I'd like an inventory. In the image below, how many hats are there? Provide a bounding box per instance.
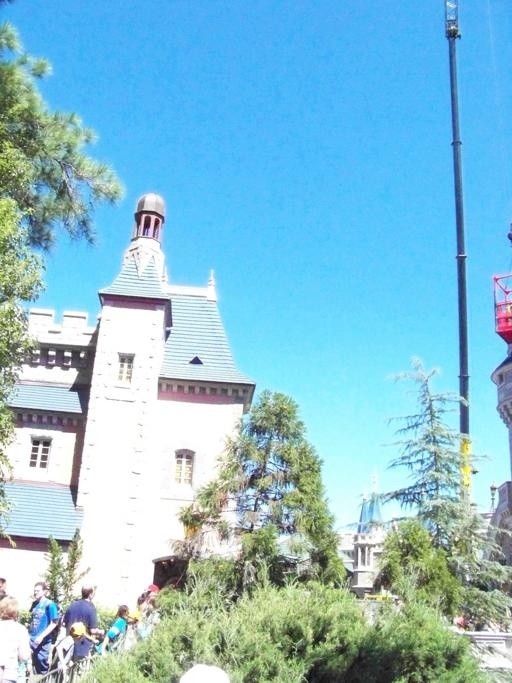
[69,623,87,637]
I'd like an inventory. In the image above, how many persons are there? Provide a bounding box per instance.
[0,574,167,683]
